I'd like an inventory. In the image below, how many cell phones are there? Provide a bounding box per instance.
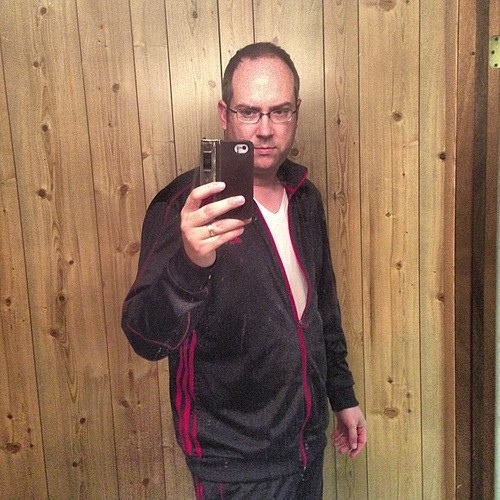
[200,137,254,221]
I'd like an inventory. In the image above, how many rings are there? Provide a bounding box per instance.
[206,222,217,238]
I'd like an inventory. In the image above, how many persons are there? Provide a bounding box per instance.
[119,42,367,499]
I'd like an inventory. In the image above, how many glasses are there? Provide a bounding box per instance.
[225,107,299,123]
